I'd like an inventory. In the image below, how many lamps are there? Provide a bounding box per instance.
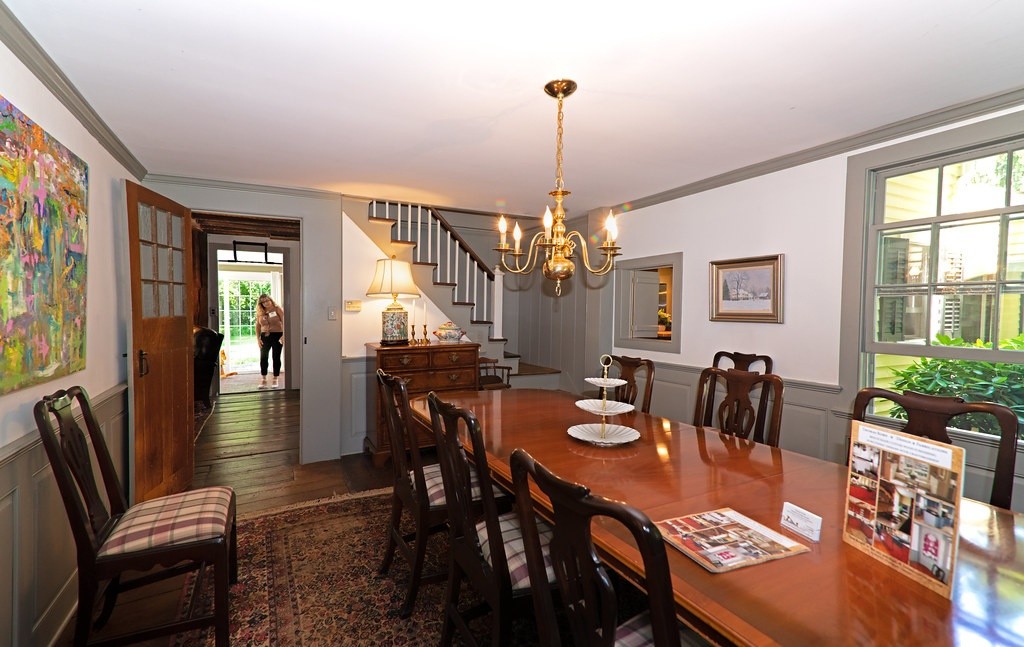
[490,79,623,299]
[366,256,421,346]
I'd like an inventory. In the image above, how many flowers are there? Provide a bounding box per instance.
[658,309,672,322]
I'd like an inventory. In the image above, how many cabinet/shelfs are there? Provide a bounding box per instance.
[365,340,479,468]
[568,356,641,447]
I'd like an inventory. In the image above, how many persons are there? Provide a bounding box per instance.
[256,294,284,389]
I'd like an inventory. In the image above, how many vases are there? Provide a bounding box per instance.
[658,324,666,332]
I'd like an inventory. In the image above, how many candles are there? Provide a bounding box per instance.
[411,299,415,325]
[423,300,427,325]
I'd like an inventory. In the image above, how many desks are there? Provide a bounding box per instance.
[402,390,1024,647]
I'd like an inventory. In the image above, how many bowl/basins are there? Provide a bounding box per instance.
[433,322,466,342]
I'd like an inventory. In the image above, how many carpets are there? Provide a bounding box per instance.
[192,400,215,443]
[173,486,492,646]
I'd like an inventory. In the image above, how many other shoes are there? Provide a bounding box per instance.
[271,379,278,388]
[258,379,268,388]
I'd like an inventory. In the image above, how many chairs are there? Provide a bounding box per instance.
[600,354,655,413]
[34,385,236,647]
[376,367,505,619]
[479,356,514,390]
[708,351,772,442]
[427,390,621,647]
[511,449,682,647]
[847,387,1020,511]
[693,368,784,449]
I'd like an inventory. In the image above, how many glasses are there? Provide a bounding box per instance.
[260,299,271,304]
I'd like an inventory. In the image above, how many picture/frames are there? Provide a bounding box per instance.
[709,253,786,324]
